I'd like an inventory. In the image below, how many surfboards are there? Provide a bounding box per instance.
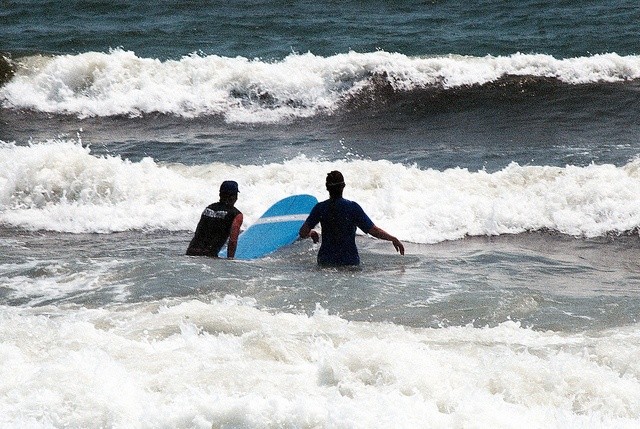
[216,194,318,260]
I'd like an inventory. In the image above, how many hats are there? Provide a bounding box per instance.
[220,181,240,197]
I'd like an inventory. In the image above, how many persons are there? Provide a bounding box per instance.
[185,181,244,259]
[299,170,405,268]
